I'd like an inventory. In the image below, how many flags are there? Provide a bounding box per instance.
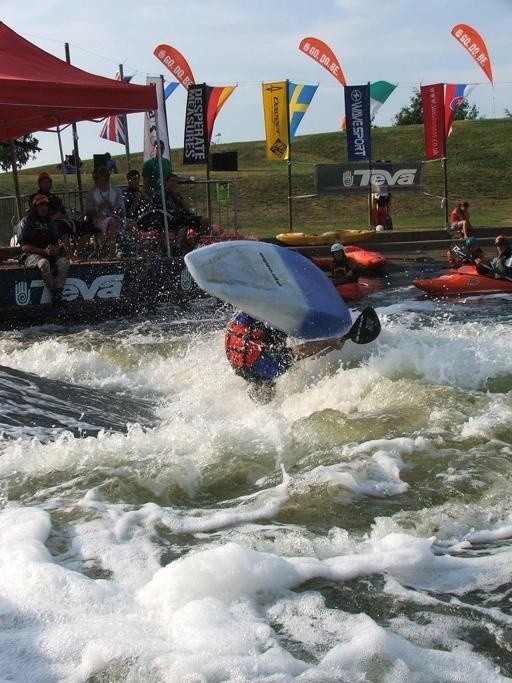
[443,83,477,140]
[99,73,132,145]
[288,80,320,144]
[342,80,398,131]
[205,85,238,145]
[164,79,180,100]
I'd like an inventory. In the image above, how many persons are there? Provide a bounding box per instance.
[224,312,344,384]
[17,193,70,309]
[371,193,392,230]
[475,234,512,282]
[67,150,83,169]
[85,139,210,260]
[326,243,358,285]
[29,172,81,264]
[447,235,485,266]
[450,201,470,239]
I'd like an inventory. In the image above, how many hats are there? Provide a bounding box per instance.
[466,236,477,247]
[330,243,345,253]
[495,234,510,247]
[32,193,49,205]
[246,380,276,406]
[38,172,52,183]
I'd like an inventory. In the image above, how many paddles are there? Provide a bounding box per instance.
[448,240,512,281]
[312,307,381,361]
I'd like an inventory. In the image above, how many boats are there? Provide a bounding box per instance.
[181,238,352,341]
[277,228,378,245]
[308,243,512,302]
[142,222,224,242]
